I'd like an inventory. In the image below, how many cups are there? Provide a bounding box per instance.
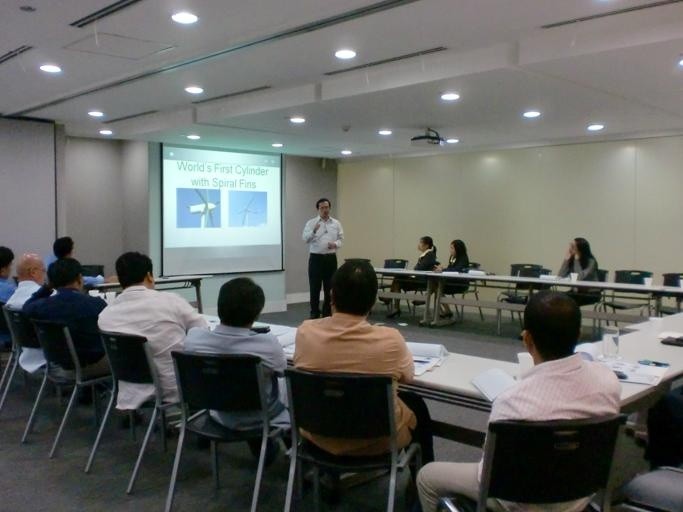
[643,277,652,286]
[680,280,683,289]
[570,272,579,281]
[87,289,116,305]
[601,325,620,358]
[517,352,532,373]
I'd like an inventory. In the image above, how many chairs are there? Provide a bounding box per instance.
[374,259,681,338]
[281,368,421,511]
[442,412,627,510]
[0,302,68,411]
[85,329,220,493]
[617,387,683,510]
[161,350,301,512]
[19,319,137,459]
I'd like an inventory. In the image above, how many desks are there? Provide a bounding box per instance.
[569,309,681,419]
[375,268,682,330]
[90,276,211,323]
[193,315,610,452]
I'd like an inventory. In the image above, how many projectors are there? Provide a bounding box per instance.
[411,136,445,146]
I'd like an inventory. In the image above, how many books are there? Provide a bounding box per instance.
[404,341,448,376]
[471,367,519,402]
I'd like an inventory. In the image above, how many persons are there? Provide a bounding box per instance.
[415,290,623,511]
[0,246,15,348]
[21,257,143,430]
[5,253,48,372]
[290,257,434,510]
[300,198,343,321]
[517,236,601,341]
[181,276,291,462]
[437,240,470,318]
[377,235,437,319]
[97,250,207,399]
[44,236,118,288]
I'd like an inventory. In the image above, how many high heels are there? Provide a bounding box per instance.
[378,297,389,305]
[439,313,453,319]
[412,300,425,305]
[386,309,400,318]
